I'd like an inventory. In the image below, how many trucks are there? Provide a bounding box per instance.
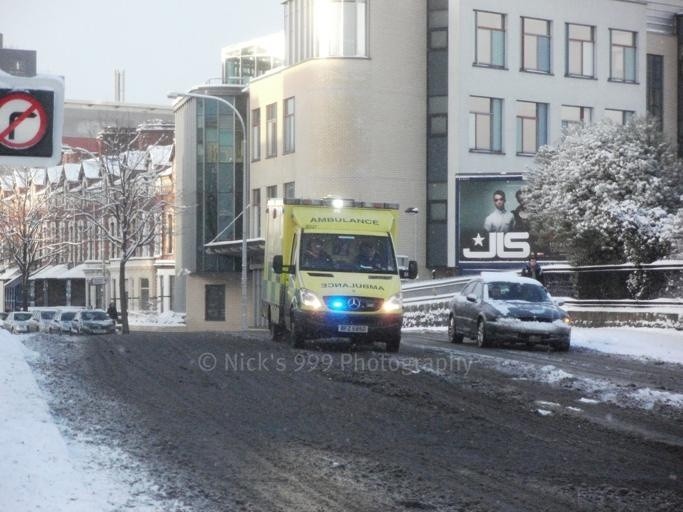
[260,197,418,353]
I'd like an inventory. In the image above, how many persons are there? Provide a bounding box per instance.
[513,187,531,232]
[522,257,548,294]
[302,239,334,271]
[353,241,385,273]
[106,301,119,334]
[484,190,516,234]
[491,286,506,299]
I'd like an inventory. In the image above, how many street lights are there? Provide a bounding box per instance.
[166,91,250,340]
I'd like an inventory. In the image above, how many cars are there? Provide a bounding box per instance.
[0,306,116,334]
[448,271,572,352]
